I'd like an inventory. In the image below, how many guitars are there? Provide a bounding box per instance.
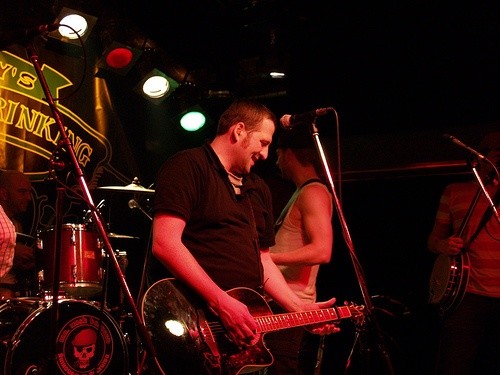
[429,173,490,311]
[142,278,364,375]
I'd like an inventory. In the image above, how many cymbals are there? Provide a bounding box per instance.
[99,181,154,192]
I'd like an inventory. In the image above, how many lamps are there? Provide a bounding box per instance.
[47,0,215,136]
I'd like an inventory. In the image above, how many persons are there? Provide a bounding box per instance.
[146,100,340,374]
[244,172,274,250]
[0,170,41,306]
[429,137,500,375]
[270,130,333,374]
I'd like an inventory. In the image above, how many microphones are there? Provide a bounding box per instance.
[15,22,59,45]
[279,107,333,131]
[128,199,152,220]
[443,133,485,160]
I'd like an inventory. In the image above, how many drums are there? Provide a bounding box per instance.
[35,223,108,295]
[1,293,132,374]
